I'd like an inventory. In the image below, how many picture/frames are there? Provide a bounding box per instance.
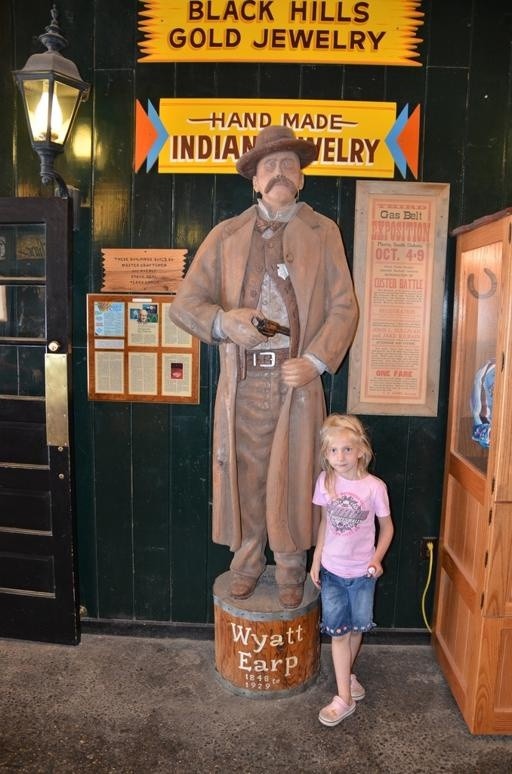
[346,179,450,417]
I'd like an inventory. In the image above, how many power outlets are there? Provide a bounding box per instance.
[420,537,437,561]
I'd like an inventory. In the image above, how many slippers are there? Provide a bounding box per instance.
[351,672,365,701]
[318,696,356,727]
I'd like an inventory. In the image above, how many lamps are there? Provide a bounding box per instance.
[11,5,92,233]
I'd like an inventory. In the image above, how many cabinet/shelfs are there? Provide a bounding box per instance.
[430,208,512,737]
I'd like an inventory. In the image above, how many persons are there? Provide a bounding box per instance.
[169,123,358,610]
[307,413,394,728]
[137,309,149,323]
